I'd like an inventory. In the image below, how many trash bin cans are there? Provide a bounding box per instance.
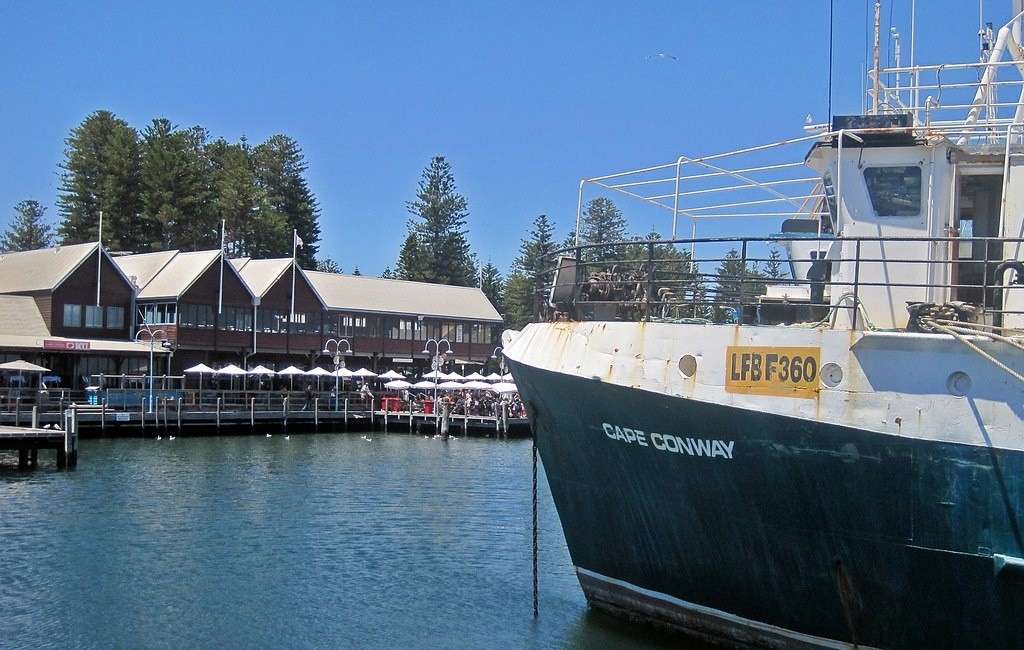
[424,400,434,414]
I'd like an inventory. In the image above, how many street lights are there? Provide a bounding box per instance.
[134,329,172,413]
[323,339,353,411]
[491,347,507,381]
[423,338,454,413]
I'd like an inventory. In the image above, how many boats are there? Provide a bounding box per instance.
[499,0,1024,650]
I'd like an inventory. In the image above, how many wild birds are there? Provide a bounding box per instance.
[645,53,679,61]
[266,434,272,437]
[485,434,489,436]
[43,424,50,430]
[361,435,372,442]
[805,114,813,125]
[157,435,161,440]
[170,435,175,440]
[424,435,429,439]
[432,434,458,440]
[284,436,290,440]
[54,424,62,431]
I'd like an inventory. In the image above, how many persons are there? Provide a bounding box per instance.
[360,382,374,411]
[399,390,525,417]
[304,385,313,411]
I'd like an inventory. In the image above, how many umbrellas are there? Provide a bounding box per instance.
[0,359,52,397]
[184,363,519,410]
